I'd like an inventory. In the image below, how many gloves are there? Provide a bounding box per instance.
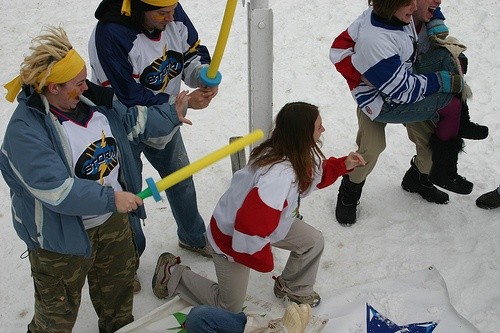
[433,71,465,96]
[427,18,449,44]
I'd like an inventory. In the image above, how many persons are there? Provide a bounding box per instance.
[87,0,218,291]
[329,0,489,227]
[476,183,500,210]
[0,23,193,332]
[152,101,366,314]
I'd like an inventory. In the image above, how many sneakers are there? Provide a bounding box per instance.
[270,276,321,309]
[129,268,141,295]
[151,252,181,300]
[176,237,213,259]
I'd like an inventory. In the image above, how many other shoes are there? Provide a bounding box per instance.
[474,185,500,211]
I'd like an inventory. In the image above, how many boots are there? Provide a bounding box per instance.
[241,302,312,333]
[400,154,449,205]
[335,174,367,228]
[429,137,474,195]
[456,92,489,140]
